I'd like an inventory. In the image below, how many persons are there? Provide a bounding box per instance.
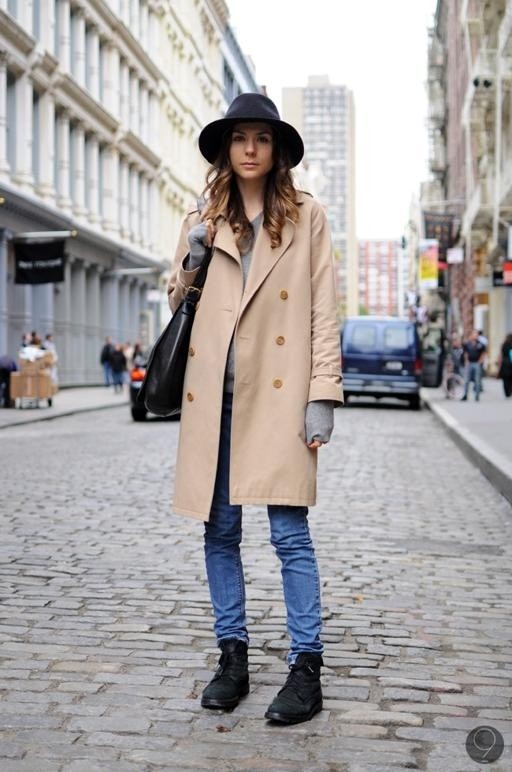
[99,335,147,395]
[164,90,347,727]
[441,328,489,402]
[0,327,59,408]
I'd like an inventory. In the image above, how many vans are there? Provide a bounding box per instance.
[335,315,447,410]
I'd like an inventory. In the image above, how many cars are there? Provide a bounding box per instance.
[130,339,182,425]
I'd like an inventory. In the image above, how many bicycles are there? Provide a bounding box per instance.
[439,336,466,401]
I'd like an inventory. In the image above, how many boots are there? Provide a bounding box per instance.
[264,651,323,723]
[200,639,249,709]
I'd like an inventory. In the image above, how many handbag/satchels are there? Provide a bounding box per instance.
[136,298,196,417]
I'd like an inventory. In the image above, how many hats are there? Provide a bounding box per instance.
[199,93,304,170]
